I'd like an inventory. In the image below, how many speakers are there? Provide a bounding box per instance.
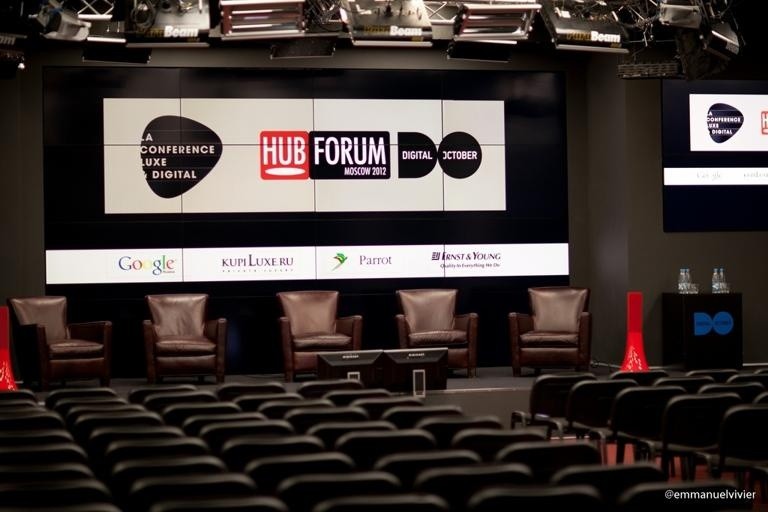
[316,349,384,388]
[384,347,450,392]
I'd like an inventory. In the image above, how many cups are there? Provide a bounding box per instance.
[678,283,729,294]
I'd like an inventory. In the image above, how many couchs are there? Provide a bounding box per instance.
[275,291,364,382]
[143,294,228,384]
[508,287,592,375]
[395,288,479,377]
[5,296,114,390]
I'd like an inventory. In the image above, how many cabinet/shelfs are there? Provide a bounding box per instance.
[661,292,743,370]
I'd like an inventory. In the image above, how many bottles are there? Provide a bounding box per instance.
[678,268,725,294]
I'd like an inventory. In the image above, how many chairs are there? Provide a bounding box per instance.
[511,369,768,501]
